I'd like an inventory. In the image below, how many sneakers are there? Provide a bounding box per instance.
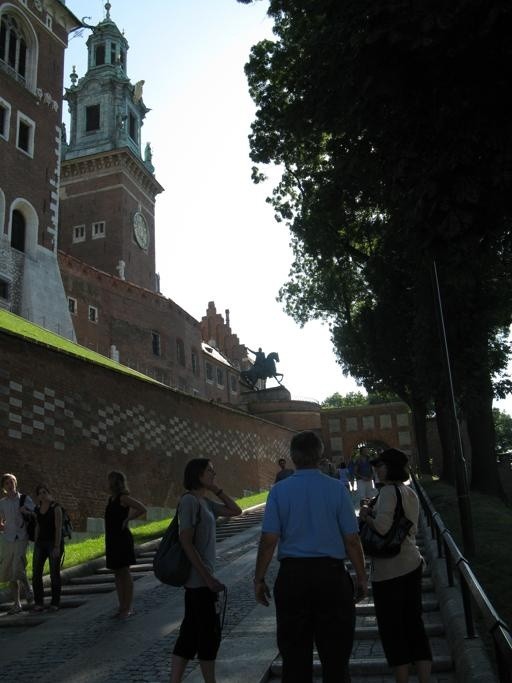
[6,584,59,615]
[110,608,137,621]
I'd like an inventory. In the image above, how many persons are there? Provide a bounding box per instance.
[253,429,367,683]
[273,456,296,485]
[31,484,64,612]
[105,469,148,619]
[320,444,385,510]
[357,447,432,683]
[1,473,35,613]
[170,458,242,683]
[246,346,266,367]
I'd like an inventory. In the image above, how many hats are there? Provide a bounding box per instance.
[370,448,408,467]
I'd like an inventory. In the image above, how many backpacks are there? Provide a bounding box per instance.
[19,493,40,542]
[51,503,73,540]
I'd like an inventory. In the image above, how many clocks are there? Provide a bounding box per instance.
[133,212,150,249]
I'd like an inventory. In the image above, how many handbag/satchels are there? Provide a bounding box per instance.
[153,514,193,587]
[357,497,414,559]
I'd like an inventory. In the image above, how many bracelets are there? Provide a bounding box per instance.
[253,577,264,584]
[30,511,33,516]
[55,542,60,547]
